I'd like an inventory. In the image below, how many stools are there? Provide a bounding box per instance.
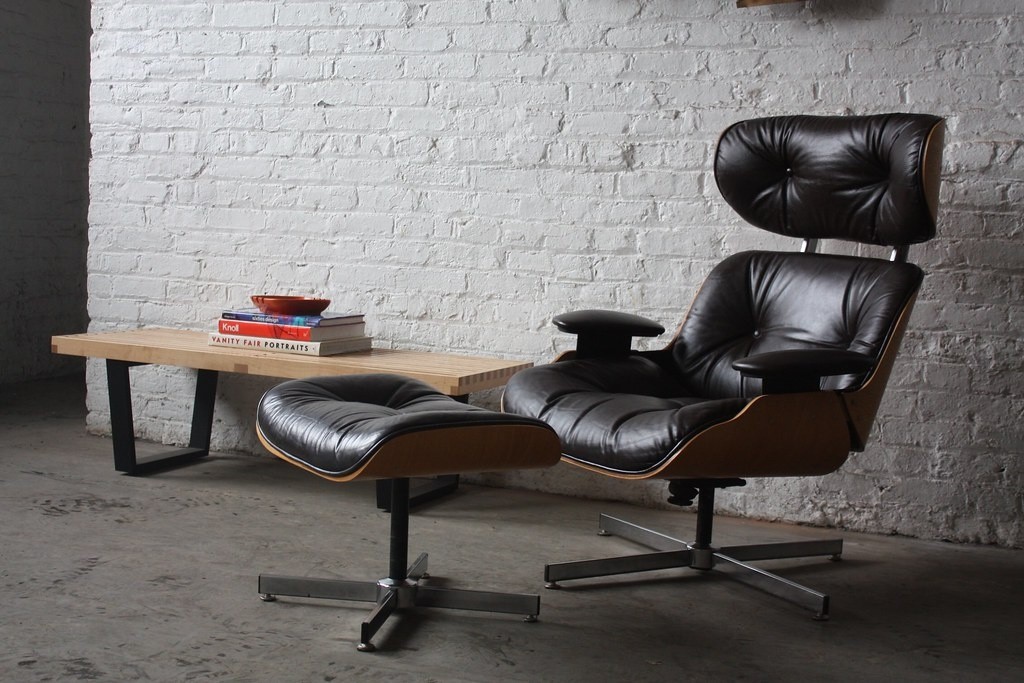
[255,372,561,650]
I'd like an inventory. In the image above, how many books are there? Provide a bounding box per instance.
[209,310,373,356]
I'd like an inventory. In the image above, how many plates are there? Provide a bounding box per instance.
[252,295,331,315]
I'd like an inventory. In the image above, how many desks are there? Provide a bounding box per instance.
[52,328,534,510]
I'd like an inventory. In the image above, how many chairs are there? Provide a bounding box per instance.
[501,112,945,620]
